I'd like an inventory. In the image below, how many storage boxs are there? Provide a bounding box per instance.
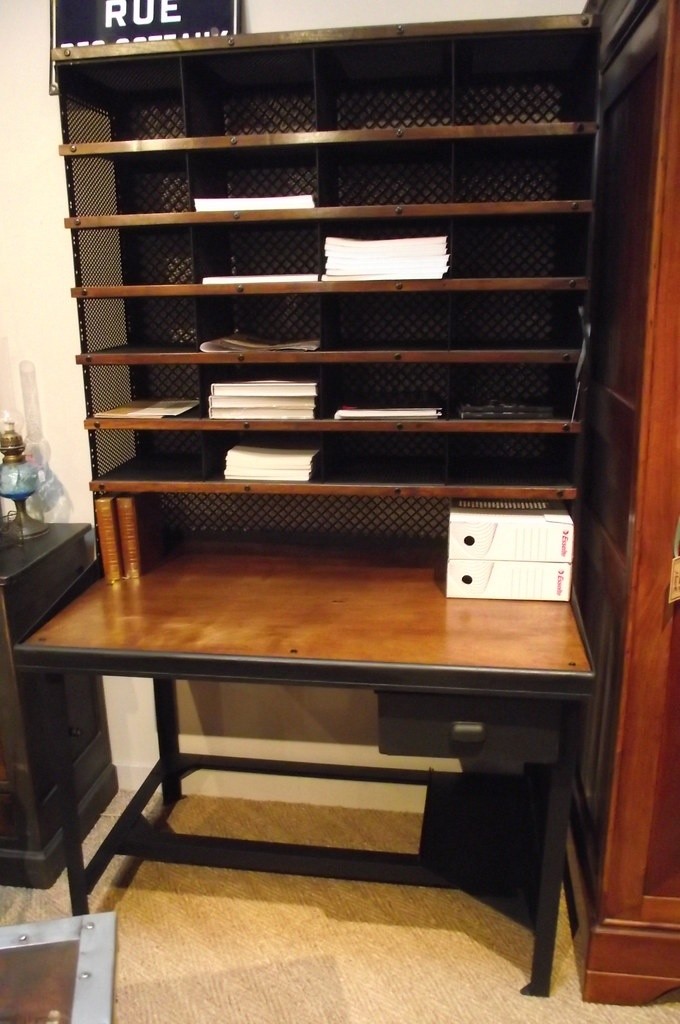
[447,501,574,601]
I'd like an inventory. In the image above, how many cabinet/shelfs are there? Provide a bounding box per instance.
[0,517,119,891]
[561,1,680,1008]
[50,12,602,546]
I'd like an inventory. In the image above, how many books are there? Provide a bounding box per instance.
[223,443,322,482]
[194,195,314,212]
[334,406,443,420]
[93,398,201,419]
[207,378,318,419]
[321,236,451,281]
[202,274,319,284]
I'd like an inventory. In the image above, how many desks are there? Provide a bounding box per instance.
[13,532,598,998]
